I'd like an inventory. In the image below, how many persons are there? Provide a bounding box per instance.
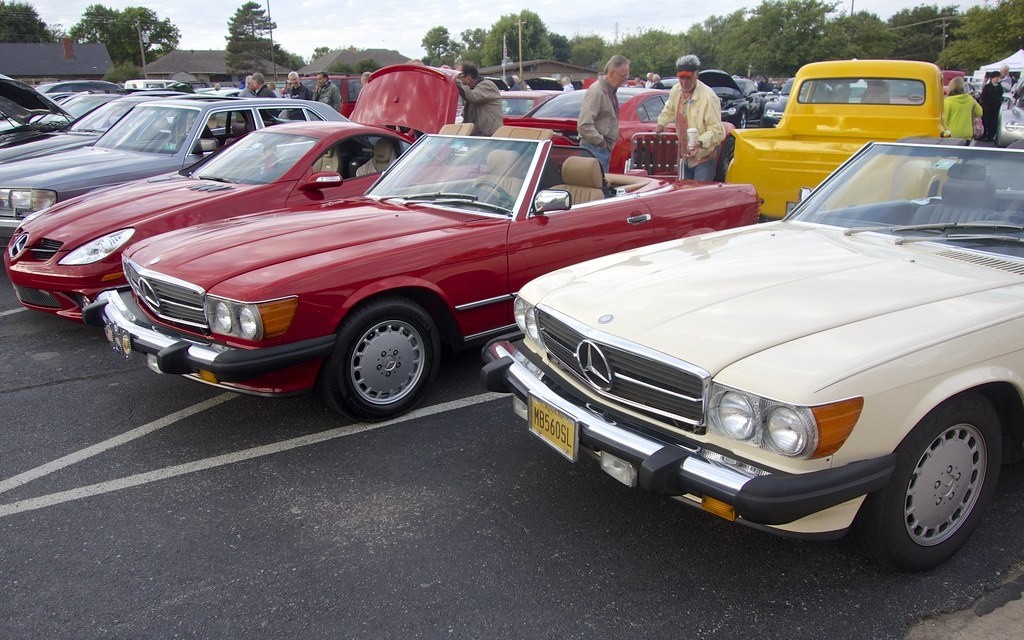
[980,66,1024,141]
[653,55,725,181]
[215,83,220,90]
[442,63,502,164]
[757,77,783,92]
[861,79,890,103]
[506,75,528,114]
[943,77,982,145]
[238,73,277,98]
[635,73,664,90]
[361,59,422,137]
[312,72,341,112]
[285,72,312,100]
[577,55,629,172]
[562,77,574,91]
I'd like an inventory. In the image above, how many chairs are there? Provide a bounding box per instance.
[356,140,396,179]
[312,139,342,177]
[226,122,245,144]
[201,124,219,149]
[473,152,522,201]
[913,174,997,234]
[546,155,606,206]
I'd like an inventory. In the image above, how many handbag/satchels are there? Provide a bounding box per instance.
[973,117,984,140]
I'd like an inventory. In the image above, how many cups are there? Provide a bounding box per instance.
[687,127,698,150]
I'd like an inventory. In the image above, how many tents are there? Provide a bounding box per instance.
[980,49,1024,79]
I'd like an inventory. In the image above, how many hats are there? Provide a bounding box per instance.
[675,65,699,77]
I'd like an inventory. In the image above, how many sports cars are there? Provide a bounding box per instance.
[81,134,764,425]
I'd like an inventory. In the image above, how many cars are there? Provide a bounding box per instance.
[478,136,1024,572]
[454,60,1024,220]
[0,64,477,326]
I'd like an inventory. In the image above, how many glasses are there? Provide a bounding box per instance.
[614,71,630,80]
